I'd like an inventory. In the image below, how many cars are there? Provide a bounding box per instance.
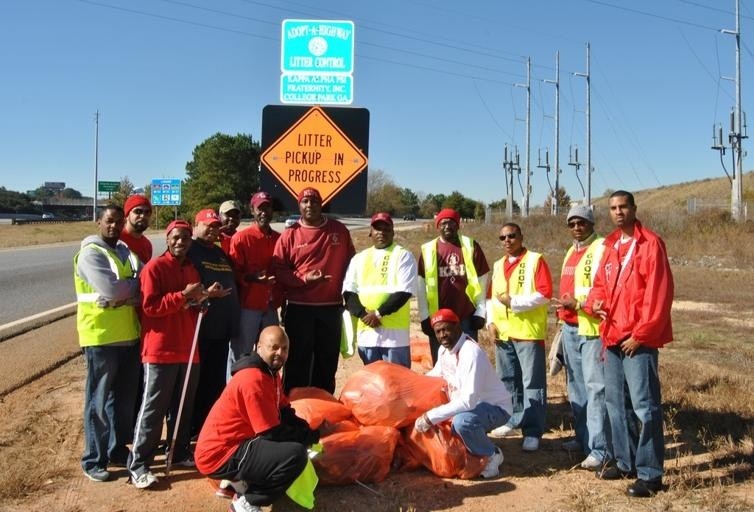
[42,212,53,219]
[285,215,300,227]
[404,213,416,221]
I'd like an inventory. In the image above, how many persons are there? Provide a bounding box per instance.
[413,306,515,480]
[413,209,492,379]
[274,184,356,397]
[580,190,675,497]
[63,190,284,496]
[486,225,553,453]
[549,205,615,470]
[340,210,420,372]
[192,322,310,512]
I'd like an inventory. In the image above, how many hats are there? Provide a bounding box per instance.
[435,207,460,229]
[166,220,193,236]
[123,194,152,217]
[298,187,322,208]
[251,192,272,207]
[219,200,241,214]
[431,308,459,328]
[566,205,595,225]
[371,212,393,225]
[195,208,222,227]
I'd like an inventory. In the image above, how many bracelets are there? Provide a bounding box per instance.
[375,308,383,321]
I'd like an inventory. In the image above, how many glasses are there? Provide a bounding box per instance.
[568,220,585,228]
[441,220,457,226]
[133,208,151,214]
[499,233,516,241]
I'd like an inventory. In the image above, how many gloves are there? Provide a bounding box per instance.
[415,415,431,433]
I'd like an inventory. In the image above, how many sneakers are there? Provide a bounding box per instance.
[167,450,195,467]
[491,425,515,436]
[216,479,249,498]
[226,493,263,512]
[628,477,663,497]
[581,455,602,470]
[522,436,539,451]
[562,440,580,451]
[108,458,127,467]
[83,465,110,482]
[602,466,632,480]
[131,470,159,489]
[480,446,504,479]
